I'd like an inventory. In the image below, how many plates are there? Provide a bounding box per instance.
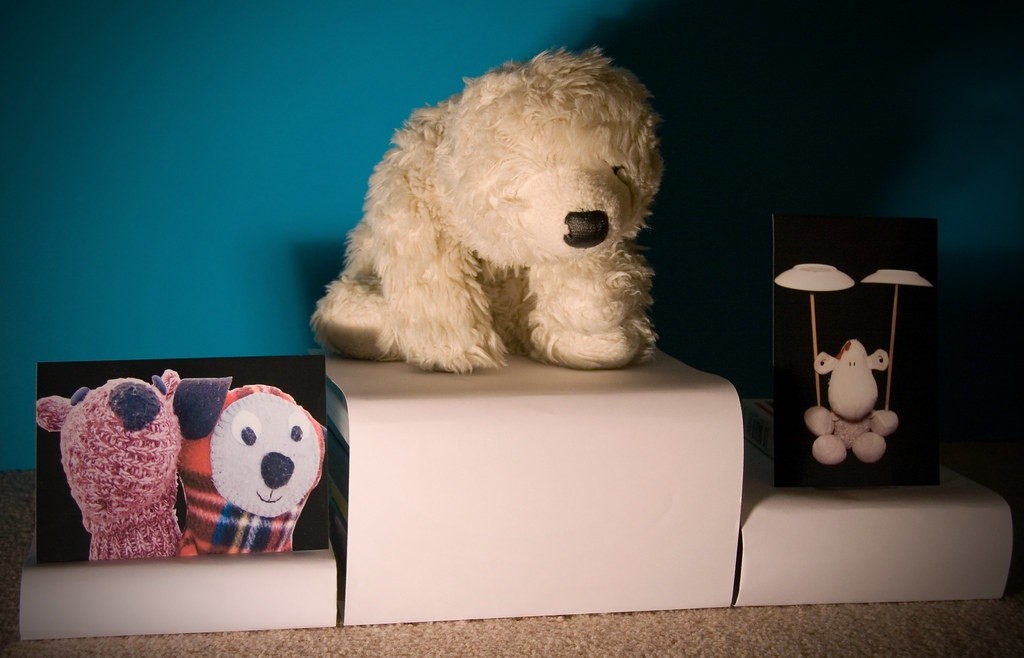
[774,263,855,292]
[860,269,933,287]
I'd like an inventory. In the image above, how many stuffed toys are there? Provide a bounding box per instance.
[310,44,666,374]
